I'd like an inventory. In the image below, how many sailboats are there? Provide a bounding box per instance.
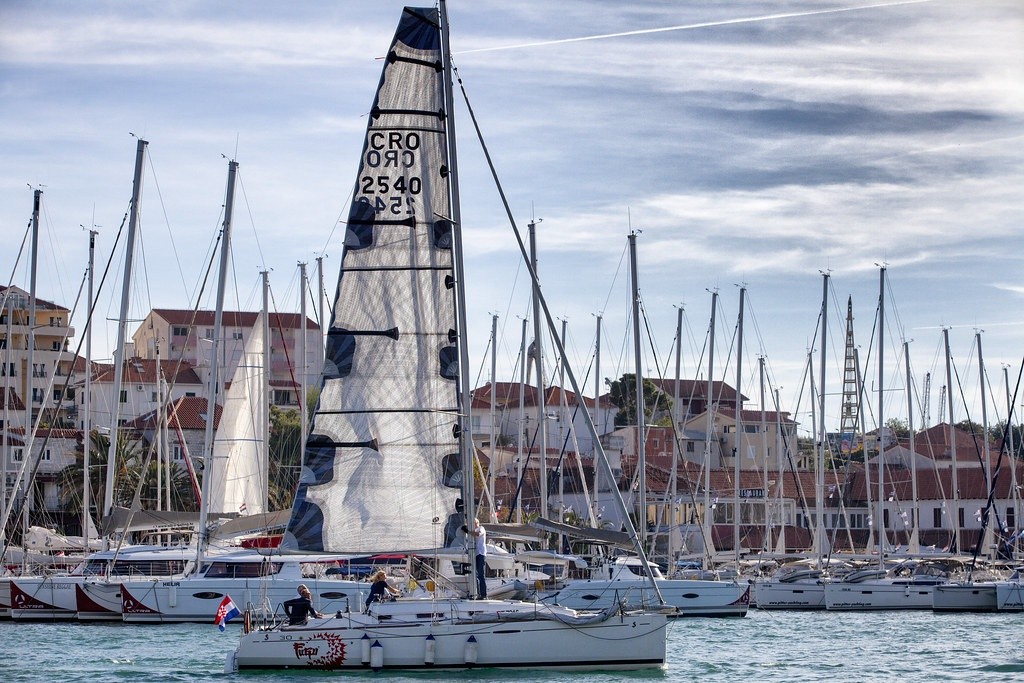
[0,0,1023,669]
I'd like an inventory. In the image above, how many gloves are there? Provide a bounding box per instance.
[462,526,468,533]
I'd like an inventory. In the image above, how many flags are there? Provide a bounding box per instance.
[214,595,239,633]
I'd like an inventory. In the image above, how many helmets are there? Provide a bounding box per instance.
[300,589,310,595]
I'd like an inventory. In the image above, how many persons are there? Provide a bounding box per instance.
[468,517,488,600]
[365,571,401,610]
[283,585,324,627]
